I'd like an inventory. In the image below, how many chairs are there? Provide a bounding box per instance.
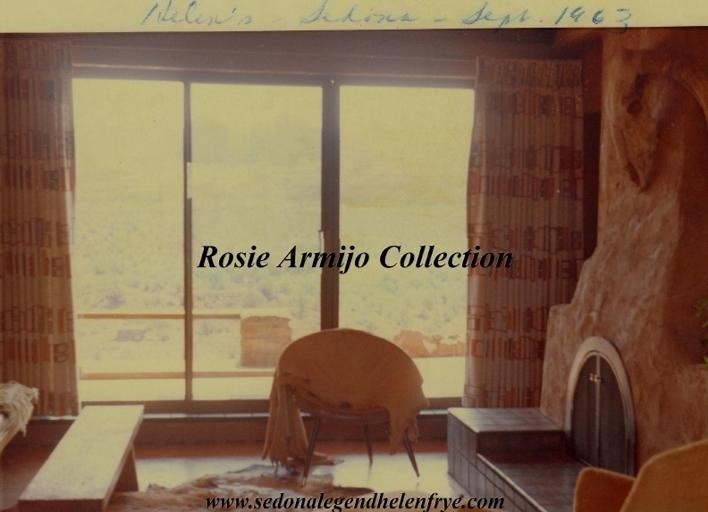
[262,327,427,477]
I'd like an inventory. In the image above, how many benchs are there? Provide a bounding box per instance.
[447,407,578,512]
[16,404,146,512]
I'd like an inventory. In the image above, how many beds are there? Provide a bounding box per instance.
[1,380,41,453]
